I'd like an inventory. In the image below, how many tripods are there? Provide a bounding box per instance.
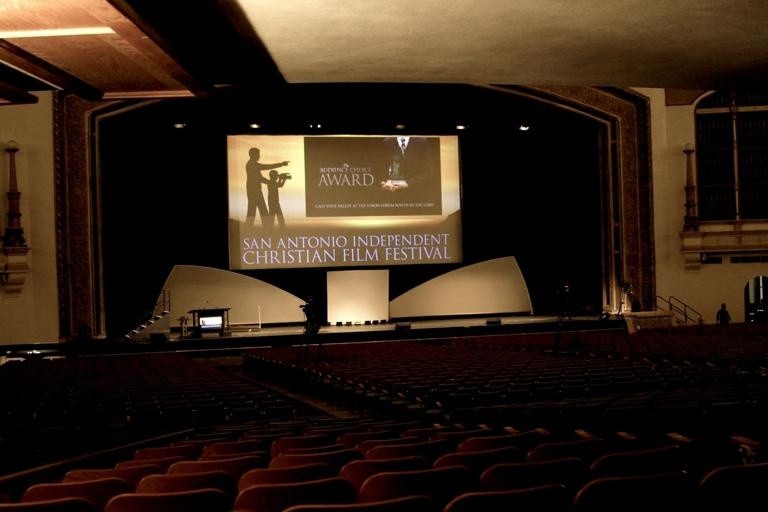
[295,324,330,368]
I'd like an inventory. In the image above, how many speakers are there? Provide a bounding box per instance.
[487,318,501,324]
[395,322,411,329]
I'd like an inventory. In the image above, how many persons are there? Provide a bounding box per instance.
[716,303,732,324]
[299,295,321,334]
[245,149,292,231]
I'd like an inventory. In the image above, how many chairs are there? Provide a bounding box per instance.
[0,323,767,512]
